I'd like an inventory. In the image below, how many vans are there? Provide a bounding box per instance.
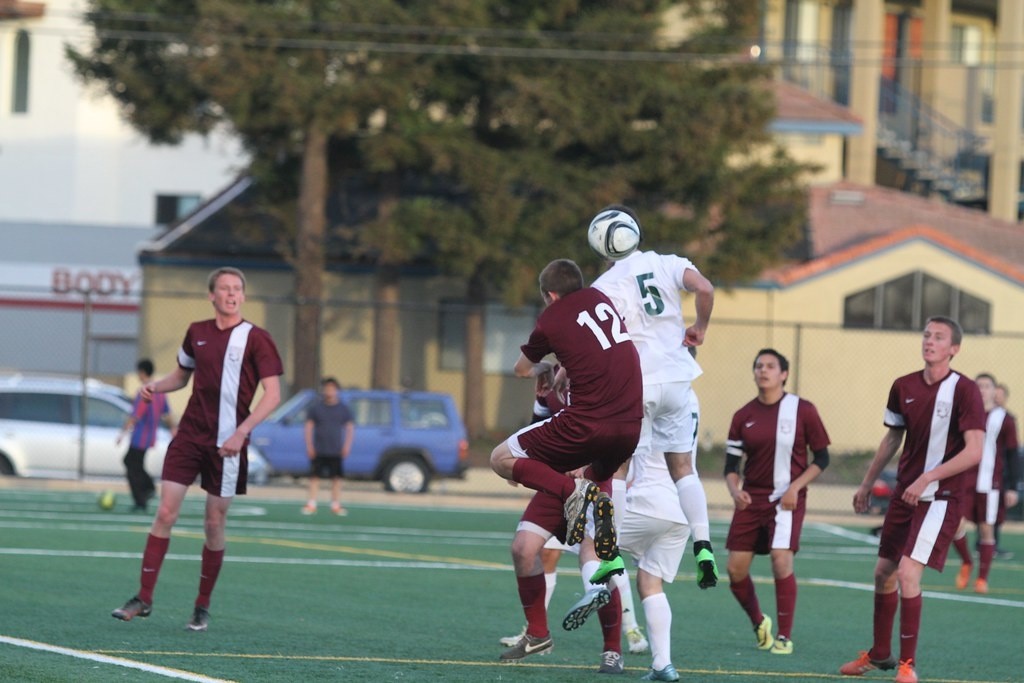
[246,385,472,496]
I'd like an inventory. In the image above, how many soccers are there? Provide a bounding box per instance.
[586,209,641,262]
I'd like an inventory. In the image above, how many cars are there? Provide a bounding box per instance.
[0,367,174,483]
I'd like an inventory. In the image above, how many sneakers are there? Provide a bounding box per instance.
[597,650,624,674]
[896,658,917,683]
[500,624,528,646]
[754,614,774,650]
[186,605,210,630]
[499,630,554,662]
[111,596,153,622]
[564,478,600,547]
[592,492,617,560]
[975,579,986,593]
[695,548,719,588]
[590,555,625,584]
[957,565,972,589]
[639,665,679,682]
[623,626,649,654]
[562,587,611,631]
[770,635,793,654]
[840,648,896,676]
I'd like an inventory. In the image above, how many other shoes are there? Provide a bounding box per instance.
[300,501,317,514]
[330,504,347,515]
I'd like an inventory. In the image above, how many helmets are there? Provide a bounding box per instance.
[587,210,640,262]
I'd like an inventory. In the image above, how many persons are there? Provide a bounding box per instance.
[500,344,700,680]
[112,266,283,628]
[590,206,717,593]
[305,379,354,517]
[725,349,831,656]
[490,259,644,561]
[953,375,1021,593]
[840,317,986,683]
[117,360,177,511]
